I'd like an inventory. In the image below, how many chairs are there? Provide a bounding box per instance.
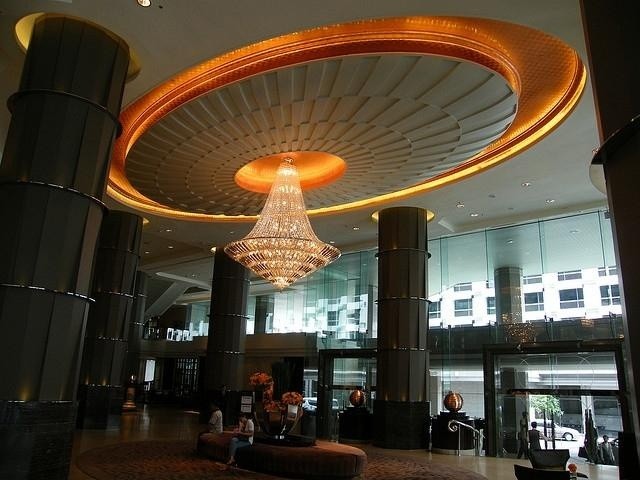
[514,448,588,480]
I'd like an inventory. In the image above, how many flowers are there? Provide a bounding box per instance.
[249,371,303,413]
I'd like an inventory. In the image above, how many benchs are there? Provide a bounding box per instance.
[198,430,367,479]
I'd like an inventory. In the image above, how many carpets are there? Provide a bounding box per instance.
[76,440,488,480]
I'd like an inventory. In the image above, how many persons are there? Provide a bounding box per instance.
[516,410,529,460]
[526,421,550,451]
[582,419,599,465]
[599,434,616,465]
[194,403,224,448]
[225,412,255,465]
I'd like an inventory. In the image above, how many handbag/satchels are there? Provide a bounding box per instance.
[237,422,251,443]
[578,446,588,459]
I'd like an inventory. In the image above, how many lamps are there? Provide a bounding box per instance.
[224,158,341,293]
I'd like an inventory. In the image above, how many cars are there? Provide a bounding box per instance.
[531,419,577,441]
[302,397,317,411]
[332,399,340,409]
